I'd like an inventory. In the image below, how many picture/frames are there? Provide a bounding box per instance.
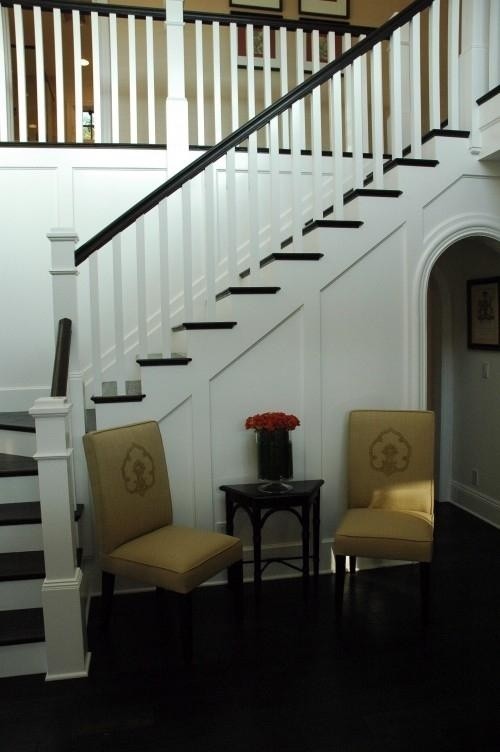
[466,276,499,352]
[228,1,352,79]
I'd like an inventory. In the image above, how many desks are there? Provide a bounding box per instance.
[216,478,326,620]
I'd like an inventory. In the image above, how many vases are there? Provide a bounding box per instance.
[257,442,296,494]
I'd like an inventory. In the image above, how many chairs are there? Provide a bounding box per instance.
[332,406,441,650]
[80,420,246,668]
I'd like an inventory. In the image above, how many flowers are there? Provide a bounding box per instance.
[244,410,302,446]
